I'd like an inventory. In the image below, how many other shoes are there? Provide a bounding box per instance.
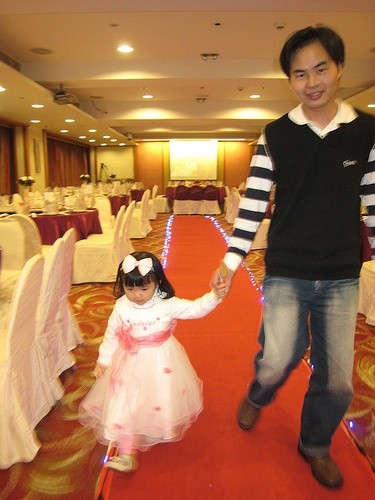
[104,453,138,474]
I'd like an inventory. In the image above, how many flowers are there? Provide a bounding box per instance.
[19,176,35,186]
[80,174,91,180]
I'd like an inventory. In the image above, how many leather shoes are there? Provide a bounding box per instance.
[238,396,260,431]
[298,440,343,488]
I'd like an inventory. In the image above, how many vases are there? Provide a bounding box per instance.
[83,179,87,187]
[22,186,28,197]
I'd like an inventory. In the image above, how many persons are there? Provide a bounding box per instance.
[211,26,375,488]
[78,250,230,472]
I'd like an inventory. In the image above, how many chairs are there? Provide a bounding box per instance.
[190,185,203,215]
[357,259,375,325]
[2,214,88,470]
[203,185,222,215]
[251,217,272,251]
[173,185,192,215]
[224,181,246,224]
[1,182,157,284]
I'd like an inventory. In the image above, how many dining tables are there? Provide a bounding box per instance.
[131,189,146,202]
[31,206,103,245]
[107,194,130,216]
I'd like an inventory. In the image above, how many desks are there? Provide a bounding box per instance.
[166,185,227,203]
